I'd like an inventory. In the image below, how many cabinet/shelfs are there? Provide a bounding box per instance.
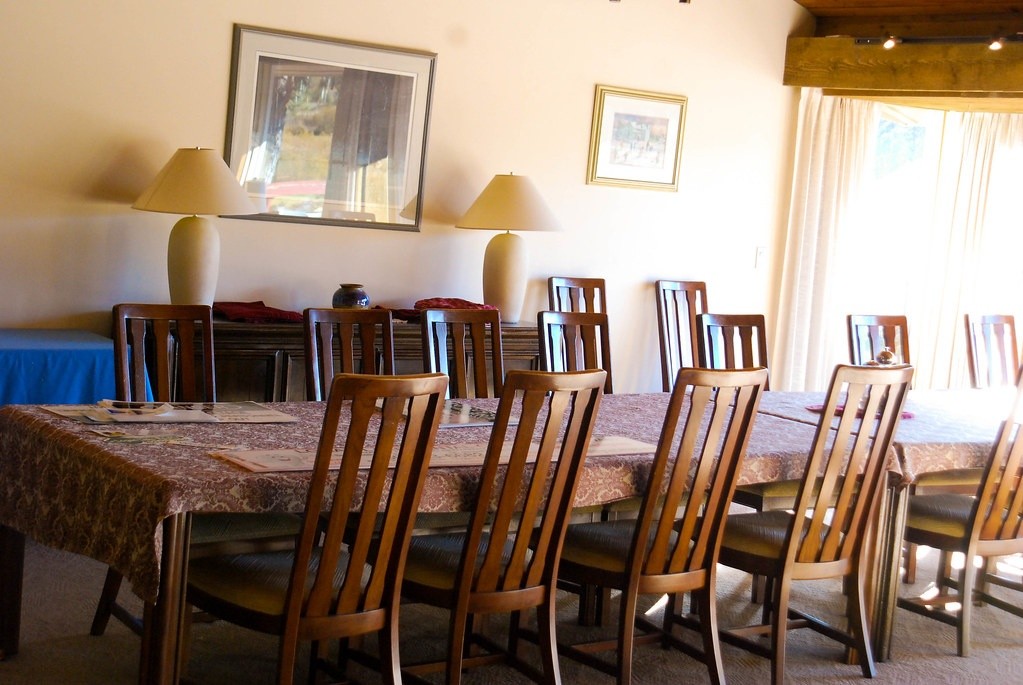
[121,322,541,402]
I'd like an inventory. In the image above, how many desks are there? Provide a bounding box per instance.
[0,401,903,685]
[0,330,154,403]
[686,391,1022,657]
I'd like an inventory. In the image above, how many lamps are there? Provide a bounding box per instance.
[133,148,261,315]
[455,174,553,325]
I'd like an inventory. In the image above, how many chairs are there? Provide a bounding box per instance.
[546,276,608,313]
[185,373,449,685]
[697,314,771,392]
[415,370,608,685]
[847,315,910,365]
[722,364,914,685]
[422,308,506,401]
[555,368,769,685]
[963,314,1021,388]
[91,304,216,641]
[900,369,1023,658]
[536,312,613,393]
[303,307,397,402]
[655,280,709,392]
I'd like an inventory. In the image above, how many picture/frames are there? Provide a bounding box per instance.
[585,84,689,192]
[222,20,439,234]
